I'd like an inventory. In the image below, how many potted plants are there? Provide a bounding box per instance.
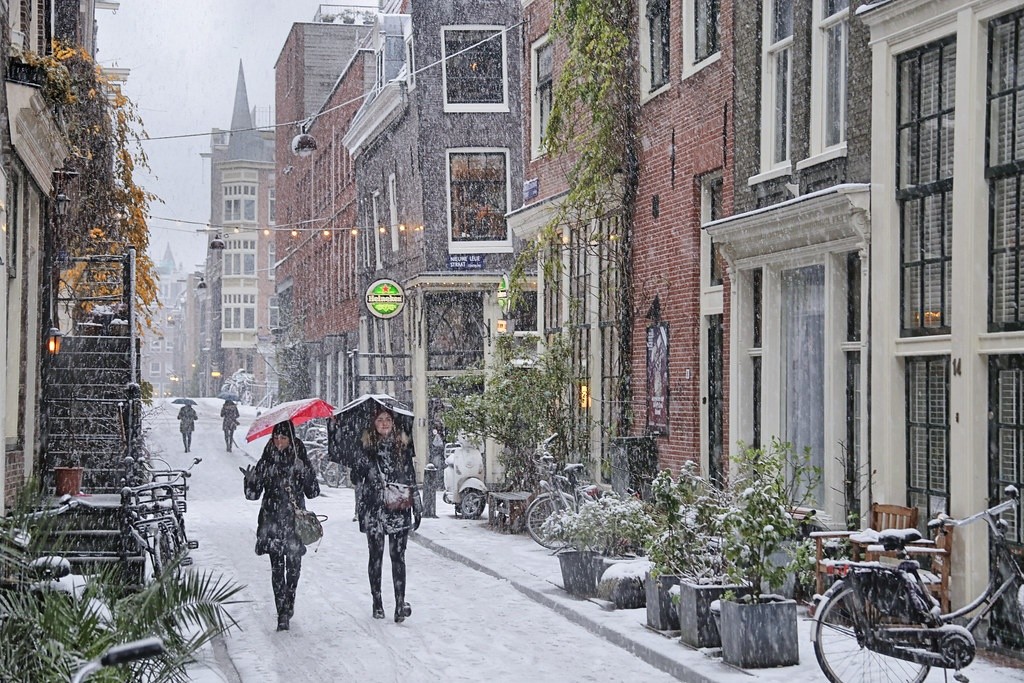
[320,9,376,24]
[538,435,820,670]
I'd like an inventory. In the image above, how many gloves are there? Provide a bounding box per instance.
[239,464,257,481]
[294,458,304,471]
[412,513,422,531]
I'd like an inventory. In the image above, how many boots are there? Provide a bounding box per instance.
[371,591,385,618]
[394,591,412,623]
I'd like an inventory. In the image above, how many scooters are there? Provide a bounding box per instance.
[441,429,488,520]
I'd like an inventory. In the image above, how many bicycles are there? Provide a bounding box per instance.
[4,494,95,615]
[106,454,203,602]
[802,486,1024,683]
[521,432,606,556]
[296,417,353,489]
[240,384,257,406]
[69,637,164,683]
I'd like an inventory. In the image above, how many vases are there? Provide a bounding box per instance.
[54,465,84,495]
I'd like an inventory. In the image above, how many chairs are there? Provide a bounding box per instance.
[859,513,953,626]
[807,501,919,626]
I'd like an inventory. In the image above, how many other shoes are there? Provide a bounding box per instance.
[278,611,294,631]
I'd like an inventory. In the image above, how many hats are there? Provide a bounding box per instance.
[273,420,295,438]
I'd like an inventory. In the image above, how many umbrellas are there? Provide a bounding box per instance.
[218,392,241,402]
[172,398,198,406]
[245,396,336,460]
[326,392,417,470]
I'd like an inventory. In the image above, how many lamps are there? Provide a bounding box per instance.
[54,182,71,221]
[196,277,207,289]
[292,123,317,157]
[42,318,64,363]
[208,235,225,249]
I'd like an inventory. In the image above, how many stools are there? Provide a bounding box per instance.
[488,491,534,534]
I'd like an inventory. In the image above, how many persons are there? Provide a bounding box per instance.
[220,399,239,453]
[239,420,321,633]
[350,408,423,623]
[177,405,198,453]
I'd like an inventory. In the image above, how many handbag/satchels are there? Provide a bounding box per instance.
[296,512,323,545]
[384,483,411,510]
